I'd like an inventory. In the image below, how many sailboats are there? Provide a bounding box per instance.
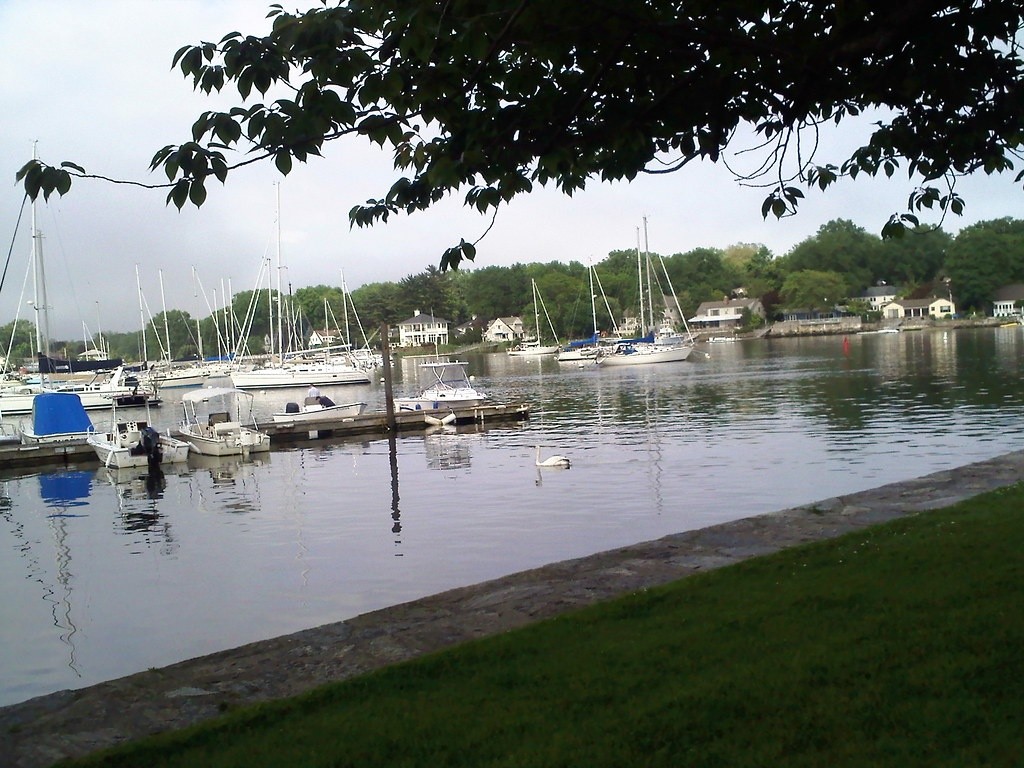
[505,278,561,356]
[0,138,394,447]
[558,216,694,365]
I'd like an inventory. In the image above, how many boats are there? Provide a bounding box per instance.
[705,335,737,343]
[271,396,369,422]
[177,385,272,457]
[86,394,189,469]
[394,305,486,413]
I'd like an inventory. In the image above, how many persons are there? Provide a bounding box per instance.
[315,396,336,409]
[308,384,320,397]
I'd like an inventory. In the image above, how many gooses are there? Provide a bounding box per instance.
[535,465,571,484]
[534,445,571,465]
